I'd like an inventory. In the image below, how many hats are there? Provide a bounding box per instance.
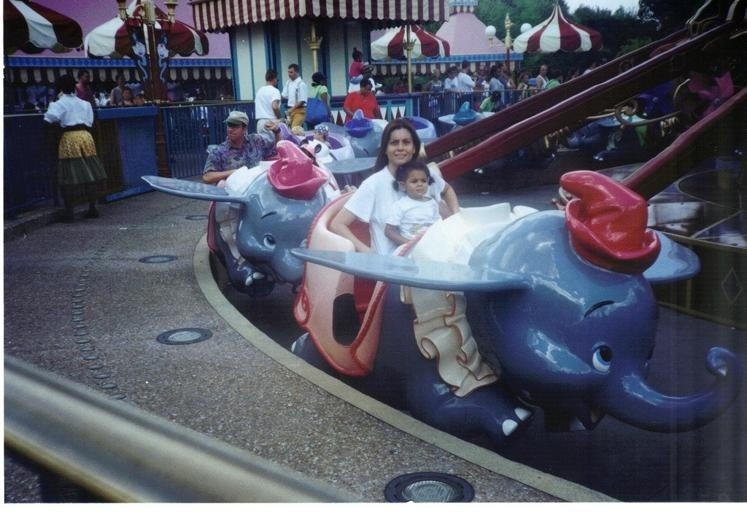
[223,111,249,127]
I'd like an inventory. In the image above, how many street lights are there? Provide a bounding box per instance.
[487,11,532,75]
[117,0,177,104]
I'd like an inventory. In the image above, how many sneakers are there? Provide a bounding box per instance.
[61,208,100,221]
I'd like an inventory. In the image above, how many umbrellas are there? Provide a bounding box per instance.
[511,3,602,55]
[371,23,450,61]
[4,0,83,108]
[84,1,209,61]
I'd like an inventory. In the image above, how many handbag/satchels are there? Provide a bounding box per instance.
[305,97,332,124]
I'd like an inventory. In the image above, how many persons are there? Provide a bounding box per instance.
[200,108,283,186]
[423,60,562,116]
[384,159,455,245]
[342,50,384,121]
[327,117,461,258]
[44,74,109,221]
[254,63,331,141]
[74,66,205,107]
